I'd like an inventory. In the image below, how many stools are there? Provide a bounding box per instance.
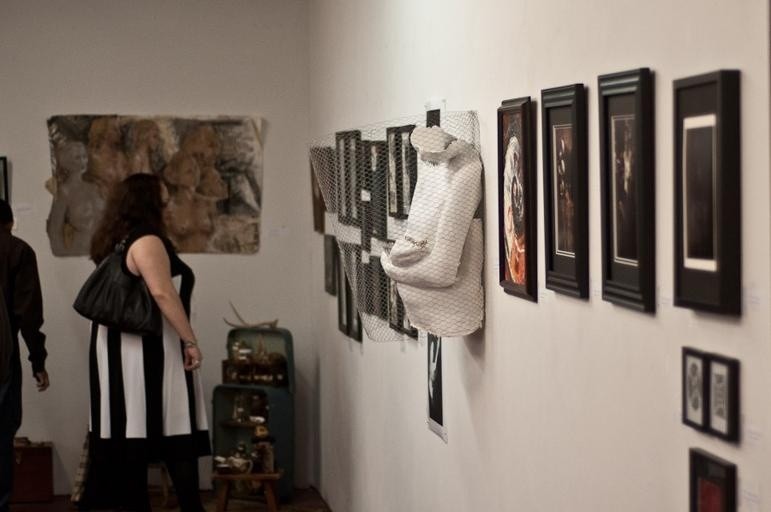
[213,466,285,512]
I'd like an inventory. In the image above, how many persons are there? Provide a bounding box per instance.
[0,199,50,512]
[74,174,212,512]
[504,153,525,283]
[47,116,262,254]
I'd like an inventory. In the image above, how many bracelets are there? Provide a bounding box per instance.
[184,341,198,347]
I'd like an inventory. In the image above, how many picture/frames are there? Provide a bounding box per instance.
[681,347,742,446]
[497,97,539,305]
[311,124,418,343]
[688,447,737,512]
[597,67,656,314]
[671,70,743,317]
[542,84,588,300]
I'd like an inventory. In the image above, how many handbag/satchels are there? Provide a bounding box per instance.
[72,234,159,337]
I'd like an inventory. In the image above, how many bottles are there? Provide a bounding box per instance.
[231,395,263,474]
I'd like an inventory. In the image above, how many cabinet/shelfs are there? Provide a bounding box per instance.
[212,326,294,493]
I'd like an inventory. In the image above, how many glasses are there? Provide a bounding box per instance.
[161,195,177,210]
